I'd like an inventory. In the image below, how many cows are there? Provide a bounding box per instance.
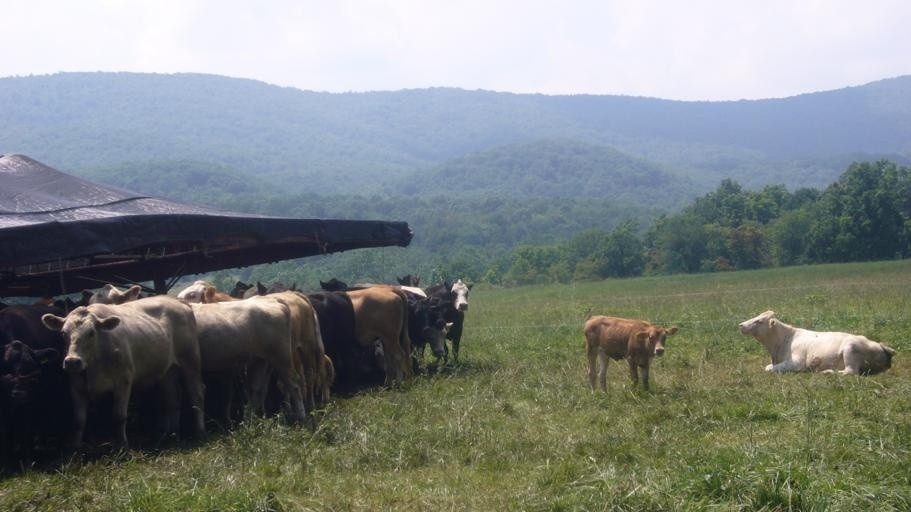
[584,315,678,393]
[0,271,475,462]
[738,309,898,376]
